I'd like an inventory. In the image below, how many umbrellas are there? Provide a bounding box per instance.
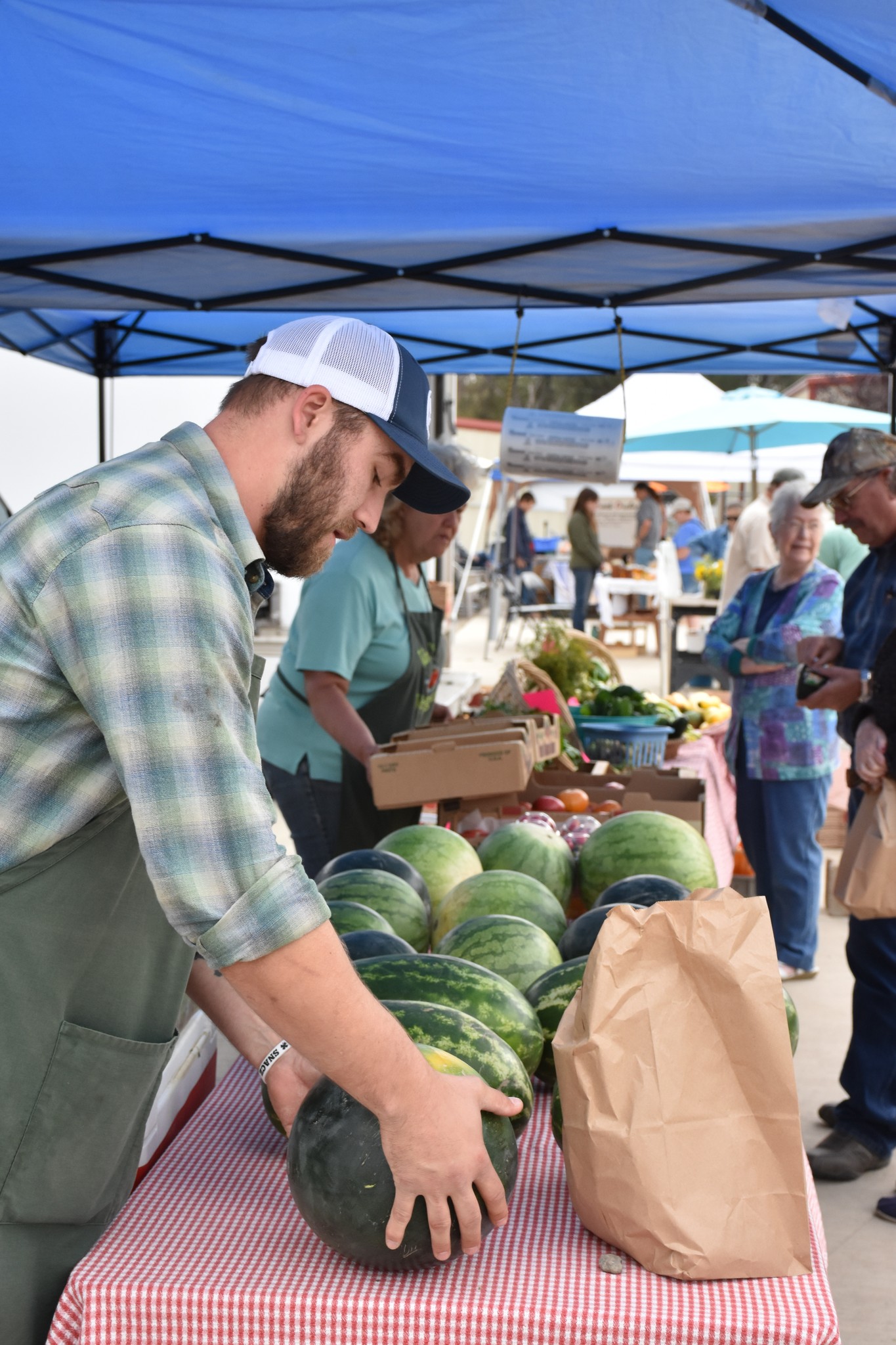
[552,371,891,498]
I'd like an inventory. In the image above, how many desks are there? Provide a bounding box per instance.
[44,1046,842,1345]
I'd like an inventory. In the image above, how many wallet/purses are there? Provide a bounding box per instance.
[795,664,827,700]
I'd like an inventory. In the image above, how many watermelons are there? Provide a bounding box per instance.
[260,811,797,1147]
[286,1041,519,1271]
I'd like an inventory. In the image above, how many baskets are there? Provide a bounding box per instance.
[487,626,623,757]
[580,722,675,770]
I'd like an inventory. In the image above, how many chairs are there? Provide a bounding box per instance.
[564,627,624,687]
[496,571,572,654]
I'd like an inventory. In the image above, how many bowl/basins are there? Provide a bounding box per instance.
[568,706,660,744]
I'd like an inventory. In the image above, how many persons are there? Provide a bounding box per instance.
[0,314,524,1342]
[254,426,896,1221]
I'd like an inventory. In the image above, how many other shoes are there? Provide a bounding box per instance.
[807,1131,892,1180]
[819,1103,838,1126]
[876,1197,896,1222]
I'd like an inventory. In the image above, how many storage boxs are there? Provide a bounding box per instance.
[368,713,708,838]
[124,1007,222,1199]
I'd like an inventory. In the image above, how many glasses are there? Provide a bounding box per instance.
[823,473,876,512]
[725,516,738,520]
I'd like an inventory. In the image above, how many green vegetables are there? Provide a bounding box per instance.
[580,685,660,717]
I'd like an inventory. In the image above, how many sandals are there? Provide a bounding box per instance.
[778,960,819,981]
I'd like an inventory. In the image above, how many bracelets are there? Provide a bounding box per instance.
[256,1040,293,1083]
[857,670,874,704]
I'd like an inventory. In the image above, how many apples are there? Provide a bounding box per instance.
[519,789,624,815]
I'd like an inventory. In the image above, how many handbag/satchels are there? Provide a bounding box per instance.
[548,883,815,1282]
[834,775,896,919]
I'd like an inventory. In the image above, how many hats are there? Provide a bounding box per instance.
[665,497,692,517]
[801,426,896,509]
[244,313,470,515]
